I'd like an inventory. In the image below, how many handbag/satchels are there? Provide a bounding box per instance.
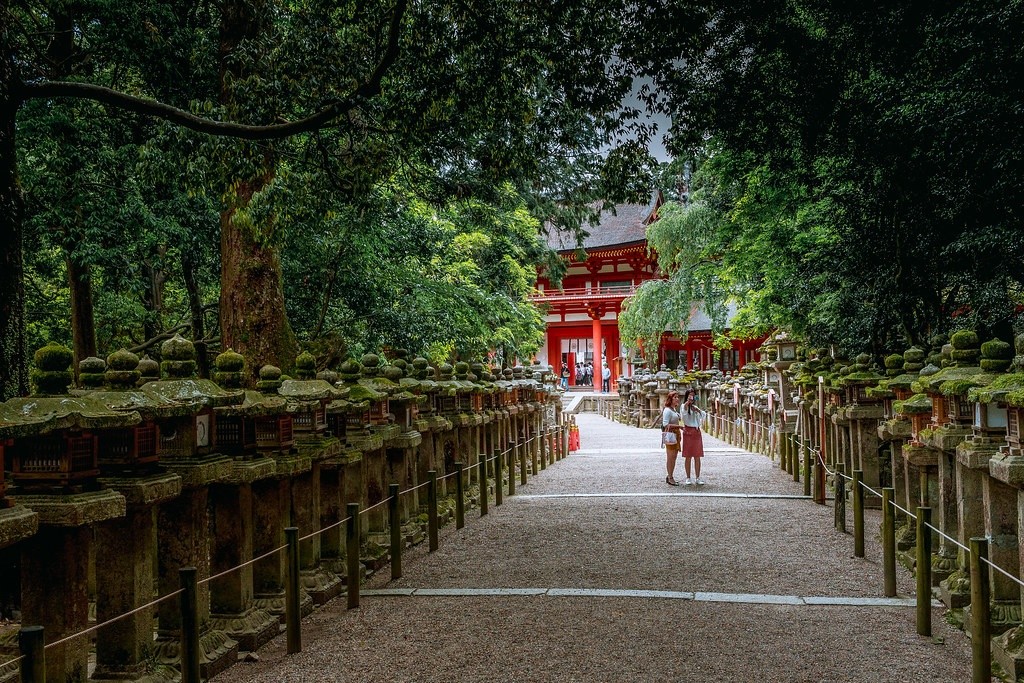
[662,426,677,445]
[563,368,570,377]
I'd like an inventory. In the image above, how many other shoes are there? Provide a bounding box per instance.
[696,478,704,484]
[686,478,691,484]
[666,476,679,486]
[578,382,593,387]
[602,390,606,394]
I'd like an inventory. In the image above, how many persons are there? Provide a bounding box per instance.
[560,361,611,394]
[661,391,686,486]
[680,389,706,484]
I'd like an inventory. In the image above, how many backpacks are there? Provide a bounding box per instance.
[577,369,582,380]
